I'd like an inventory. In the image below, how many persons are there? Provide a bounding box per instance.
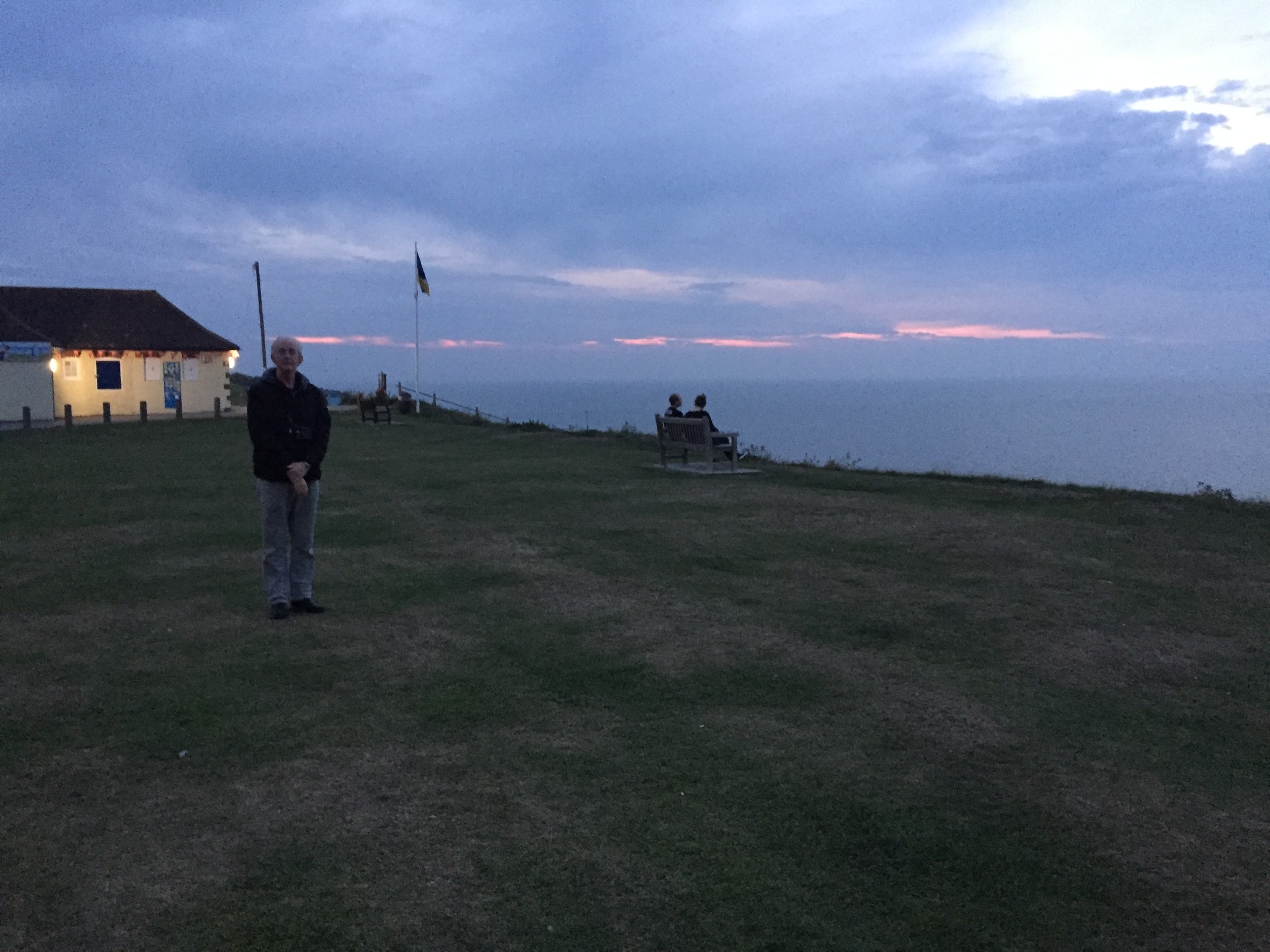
[664,393,721,463]
[247,337,330,620]
[684,395,748,462]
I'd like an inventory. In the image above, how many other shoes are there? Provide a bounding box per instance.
[267,602,289,618]
[290,597,324,613]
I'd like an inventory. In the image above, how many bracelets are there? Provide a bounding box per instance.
[303,461,312,468]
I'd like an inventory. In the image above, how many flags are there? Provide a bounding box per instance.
[416,252,430,296]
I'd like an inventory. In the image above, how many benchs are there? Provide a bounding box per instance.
[655,414,739,472]
[360,397,391,424]
